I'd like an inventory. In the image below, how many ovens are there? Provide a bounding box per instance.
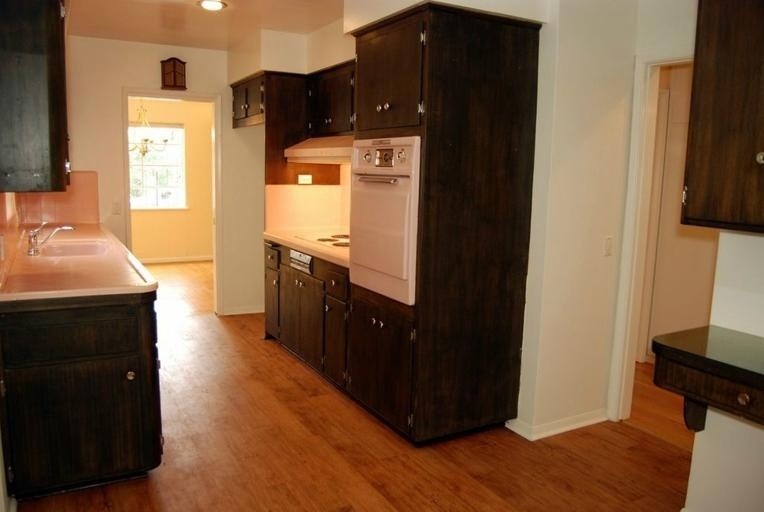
[348,135,423,306]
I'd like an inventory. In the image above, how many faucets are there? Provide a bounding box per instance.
[25,220,79,256]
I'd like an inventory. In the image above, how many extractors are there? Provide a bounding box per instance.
[283,135,353,169]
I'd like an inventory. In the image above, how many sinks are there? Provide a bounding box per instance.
[38,239,111,256]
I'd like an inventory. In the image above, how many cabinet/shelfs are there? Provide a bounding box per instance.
[231,74,304,129]
[306,69,354,137]
[653,357,763,427]
[678,0,762,236]
[263,242,349,394]
[351,0,543,447]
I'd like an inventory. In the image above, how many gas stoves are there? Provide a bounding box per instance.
[264,212,361,268]
